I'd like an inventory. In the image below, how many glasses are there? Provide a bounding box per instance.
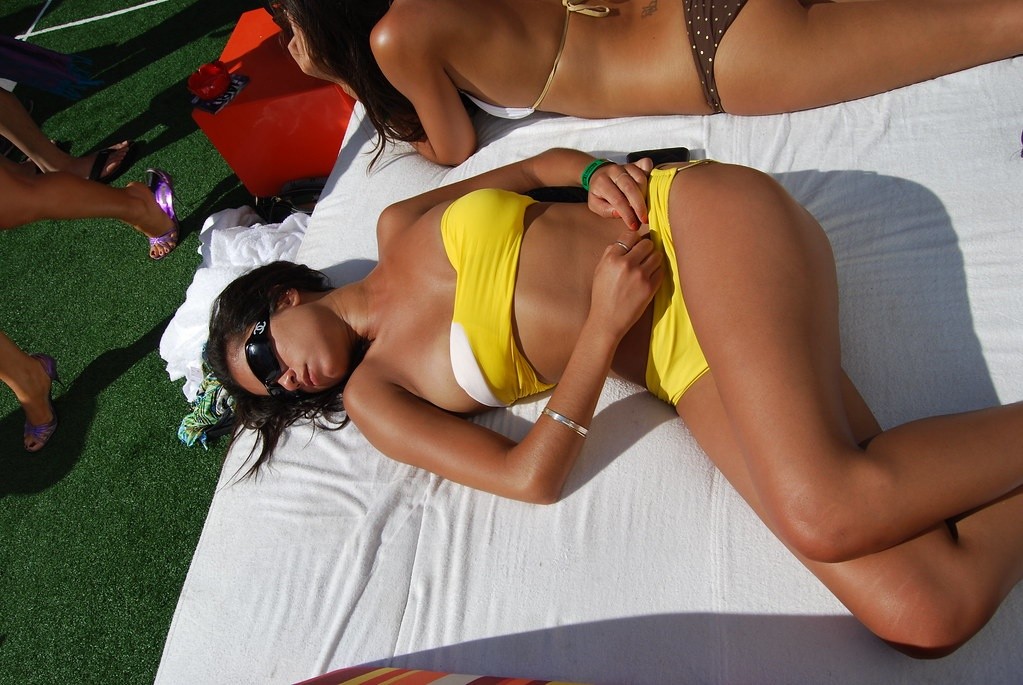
[244,309,297,409]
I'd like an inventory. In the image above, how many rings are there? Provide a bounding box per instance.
[615,173,628,184]
[616,242,628,251]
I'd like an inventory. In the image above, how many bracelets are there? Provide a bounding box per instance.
[582,158,618,190]
[541,408,588,439]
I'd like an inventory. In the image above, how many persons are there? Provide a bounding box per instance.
[264,0,1023,167]
[0,81,183,453]
[204,147,1023,660]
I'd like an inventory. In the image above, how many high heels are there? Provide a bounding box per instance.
[146,169,184,260]
[24,354,64,452]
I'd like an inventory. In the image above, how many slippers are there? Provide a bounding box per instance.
[87,142,137,185]
